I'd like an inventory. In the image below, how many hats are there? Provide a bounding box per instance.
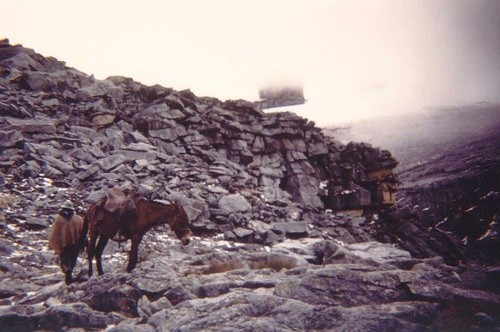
[61,199,75,210]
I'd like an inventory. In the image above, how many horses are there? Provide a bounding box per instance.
[82,191,192,278]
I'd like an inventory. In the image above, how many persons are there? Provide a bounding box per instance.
[48,199,88,285]
